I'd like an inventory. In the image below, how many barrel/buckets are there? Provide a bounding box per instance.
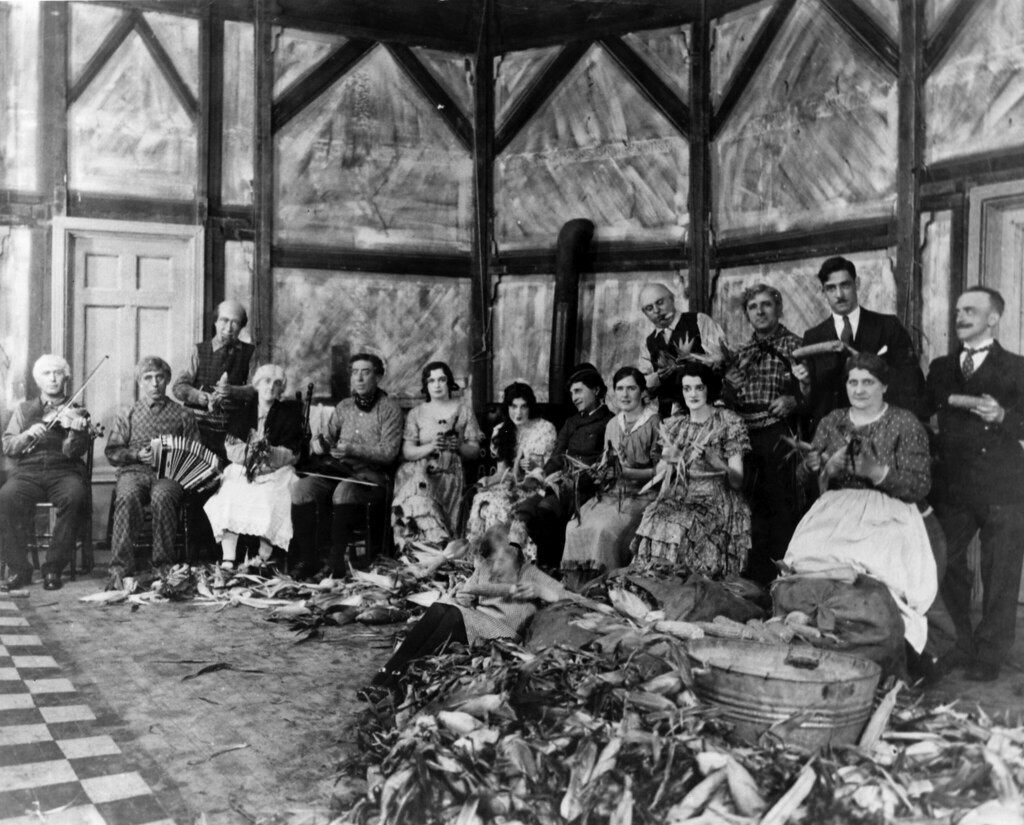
[687,640,882,752]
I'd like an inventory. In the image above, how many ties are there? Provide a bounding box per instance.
[665,328,672,345]
[962,346,994,381]
[219,344,231,366]
[841,315,854,348]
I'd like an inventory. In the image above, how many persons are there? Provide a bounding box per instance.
[559,366,662,577]
[206,358,316,589]
[356,520,581,704]
[785,257,934,433]
[390,363,481,552]
[779,351,958,683]
[520,361,614,573]
[630,283,731,420]
[106,356,201,591]
[291,353,404,576]
[467,377,561,548]
[0,355,91,587]
[719,283,803,591]
[174,299,261,566]
[626,363,750,574]
[923,286,1024,685]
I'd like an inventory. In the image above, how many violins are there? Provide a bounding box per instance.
[41,402,107,442]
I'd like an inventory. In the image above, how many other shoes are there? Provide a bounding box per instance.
[356,665,402,702]
[965,660,998,681]
[939,647,968,669]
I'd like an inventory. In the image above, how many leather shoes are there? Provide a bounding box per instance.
[43,573,62,590]
[0,574,26,592]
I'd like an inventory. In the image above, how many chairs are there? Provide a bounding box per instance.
[0,437,97,582]
[107,488,193,564]
